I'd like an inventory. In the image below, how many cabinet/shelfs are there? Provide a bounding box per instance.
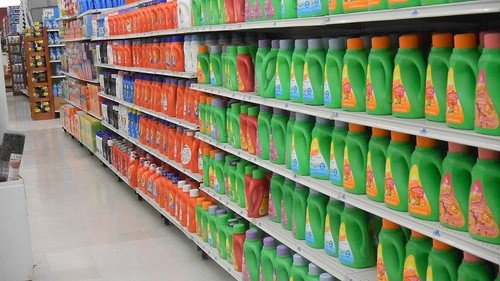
[0,0,499,281]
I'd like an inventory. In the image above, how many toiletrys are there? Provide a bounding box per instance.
[22,1,499,281]
